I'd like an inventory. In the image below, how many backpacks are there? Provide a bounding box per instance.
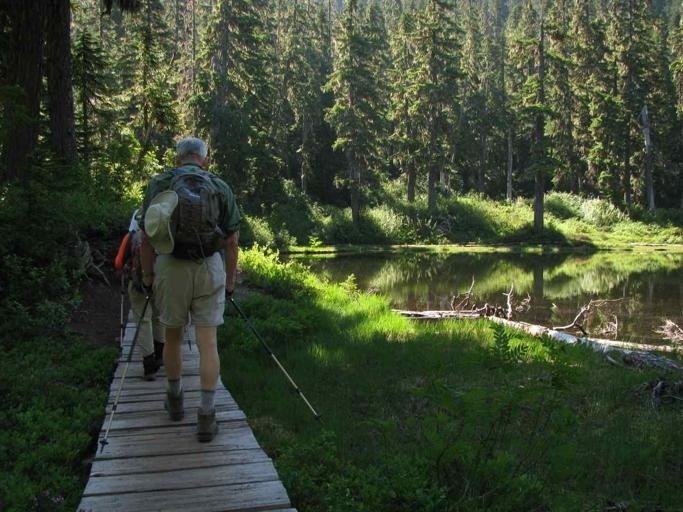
[153,168,229,263]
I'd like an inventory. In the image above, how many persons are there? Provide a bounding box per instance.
[114,206,165,382]
[137,138,241,443]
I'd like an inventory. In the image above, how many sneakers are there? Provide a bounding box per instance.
[154,351,164,367]
[164,388,185,423]
[194,407,219,443]
[143,353,159,382]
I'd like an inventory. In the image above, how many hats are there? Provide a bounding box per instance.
[143,189,180,257]
